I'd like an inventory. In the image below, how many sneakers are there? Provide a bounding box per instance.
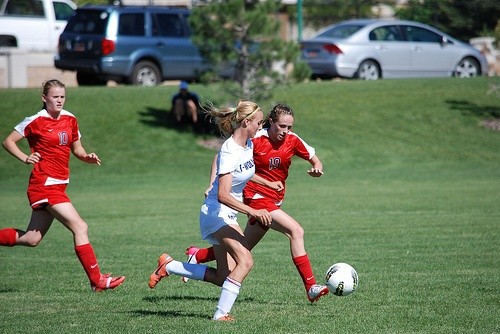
[217,316,234,321]
[91,273,125,291]
[307,284,328,302]
[149,253,173,289]
[182,246,200,283]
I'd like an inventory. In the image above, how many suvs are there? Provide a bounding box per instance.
[54,4,260,87]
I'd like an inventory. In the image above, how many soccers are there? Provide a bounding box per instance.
[325,262,359,297]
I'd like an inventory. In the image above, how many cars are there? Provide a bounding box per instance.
[0,0,77,52]
[300,18,488,82]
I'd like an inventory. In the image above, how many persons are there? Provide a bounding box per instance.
[148,99,284,321]
[0,79,125,292]
[171,81,206,133]
[182,104,329,303]
